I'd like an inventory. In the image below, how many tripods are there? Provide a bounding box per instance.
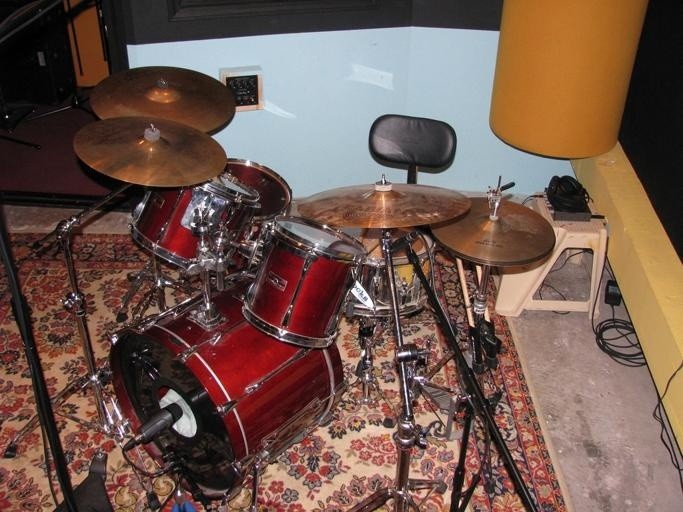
[340,320,394,413]
[116,250,199,327]
[3,183,161,510]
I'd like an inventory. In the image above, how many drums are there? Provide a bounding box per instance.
[128,174,261,272]
[336,230,437,317]
[108,266,345,501]
[242,216,368,350]
[222,158,294,275]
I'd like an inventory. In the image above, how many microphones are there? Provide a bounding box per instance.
[124,403,183,452]
[390,231,418,253]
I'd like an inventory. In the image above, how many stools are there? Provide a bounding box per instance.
[497,194,606,322]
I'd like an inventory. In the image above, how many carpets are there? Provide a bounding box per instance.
[2,225,567,512]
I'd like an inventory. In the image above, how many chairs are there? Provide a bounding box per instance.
[368,116,457,186]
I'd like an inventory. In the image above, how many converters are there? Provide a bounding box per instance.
[604,279,621,305]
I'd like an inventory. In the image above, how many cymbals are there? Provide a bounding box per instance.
[430,198,555,269]
[90,66,234,134]
[71,116,228,189]
[296,183,470,229]
